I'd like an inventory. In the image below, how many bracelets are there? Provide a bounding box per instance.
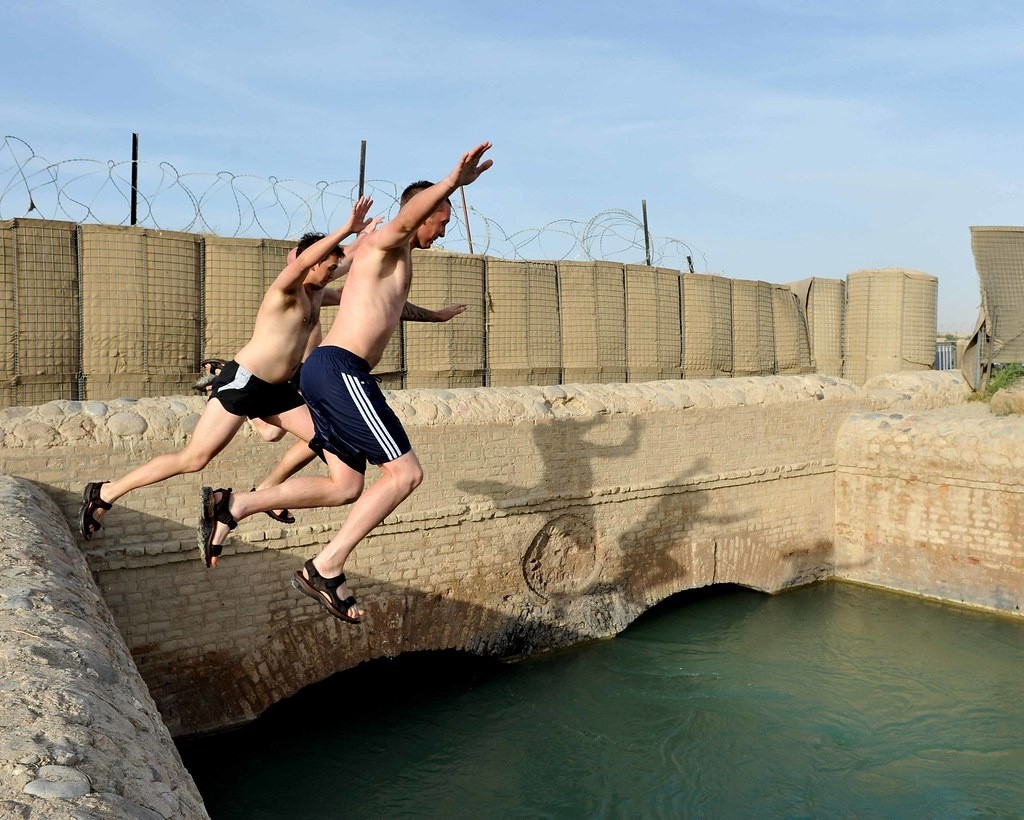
[358,230,368,236]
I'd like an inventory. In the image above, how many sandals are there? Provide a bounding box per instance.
[193,484,238,571]
[250,486,296,525]
[77,479,113,541]
[289,553,363,625]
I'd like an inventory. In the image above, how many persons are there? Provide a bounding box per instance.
[76,194,386,543]
[190,210,387,527]
[198,140,494,625]
